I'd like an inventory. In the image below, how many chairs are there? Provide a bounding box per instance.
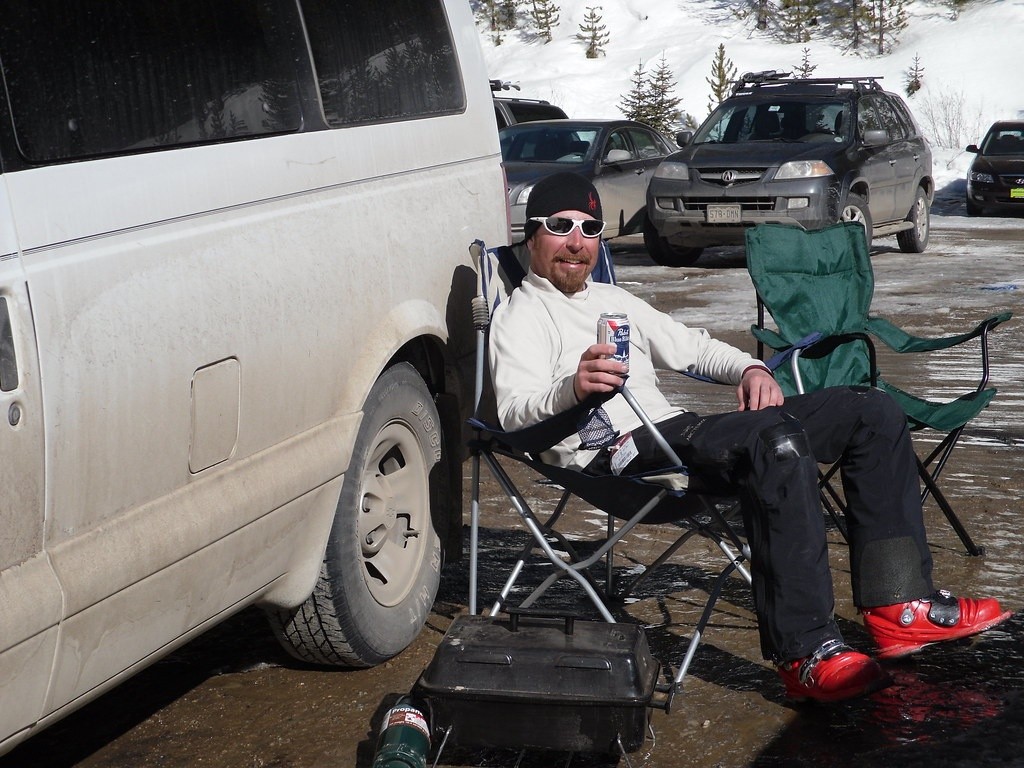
[468,238,753,697]
[744,222,1014,566]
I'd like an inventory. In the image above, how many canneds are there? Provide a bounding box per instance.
[597,311,630,375]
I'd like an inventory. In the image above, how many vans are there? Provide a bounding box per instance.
[0,0,518,755]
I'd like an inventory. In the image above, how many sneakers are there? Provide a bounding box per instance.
[861,593,1012,660]
[778,639,884,701]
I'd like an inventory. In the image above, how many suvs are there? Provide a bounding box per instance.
[646,68,935,265]
[492,96,582,162]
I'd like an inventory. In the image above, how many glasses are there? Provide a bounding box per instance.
[526,216,606,238]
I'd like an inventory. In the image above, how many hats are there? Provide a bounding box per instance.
[524,173,603,243]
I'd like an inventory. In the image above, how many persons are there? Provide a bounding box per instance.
[483,172,1013,709]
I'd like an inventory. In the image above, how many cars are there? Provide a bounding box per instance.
[963,120,1024,218]
[499,118,682,245]
